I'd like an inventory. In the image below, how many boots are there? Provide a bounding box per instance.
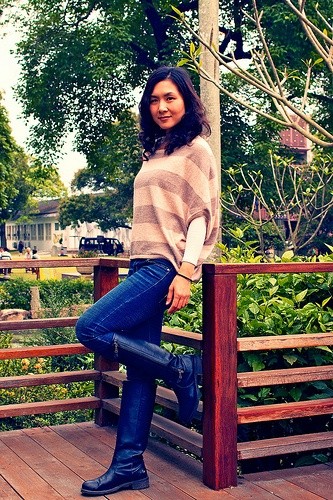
[103,330,202,424]
[80,380,158,494]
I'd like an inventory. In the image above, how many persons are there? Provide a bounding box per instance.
[76,66,220,495]
[1,240,124,273]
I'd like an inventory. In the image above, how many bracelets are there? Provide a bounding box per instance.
[176,272,194,282]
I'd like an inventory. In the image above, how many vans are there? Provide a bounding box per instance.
[69,236,124,257]
